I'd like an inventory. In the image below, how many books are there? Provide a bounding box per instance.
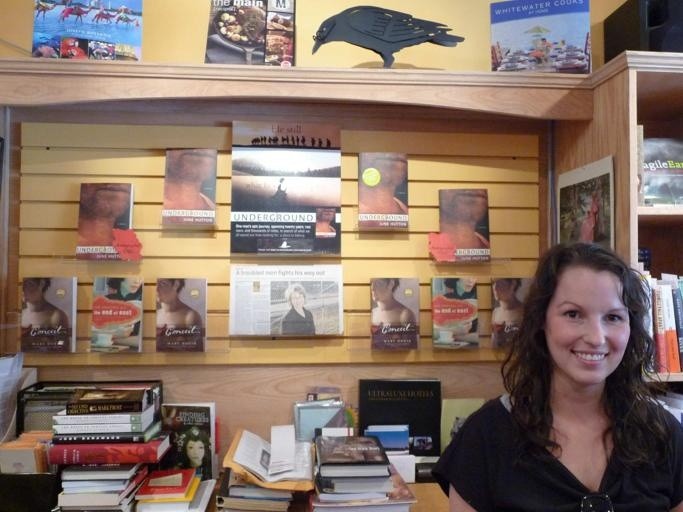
[77,182,133,261]
[357,376,442,459]
[2,381,219,512]
[637,244,682,378]
[229,112,341,258]
[162,146,219,231]
[358,150,535,354]
[216,394,418,512]
[22,272,209,352]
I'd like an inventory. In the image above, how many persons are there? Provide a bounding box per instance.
[281,282,315,335]
[447,243,682,512]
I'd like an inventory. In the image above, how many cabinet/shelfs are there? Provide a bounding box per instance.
[1,60,594,511]
[548,47,683,385]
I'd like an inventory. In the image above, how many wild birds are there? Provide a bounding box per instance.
[312,5,466,68]
